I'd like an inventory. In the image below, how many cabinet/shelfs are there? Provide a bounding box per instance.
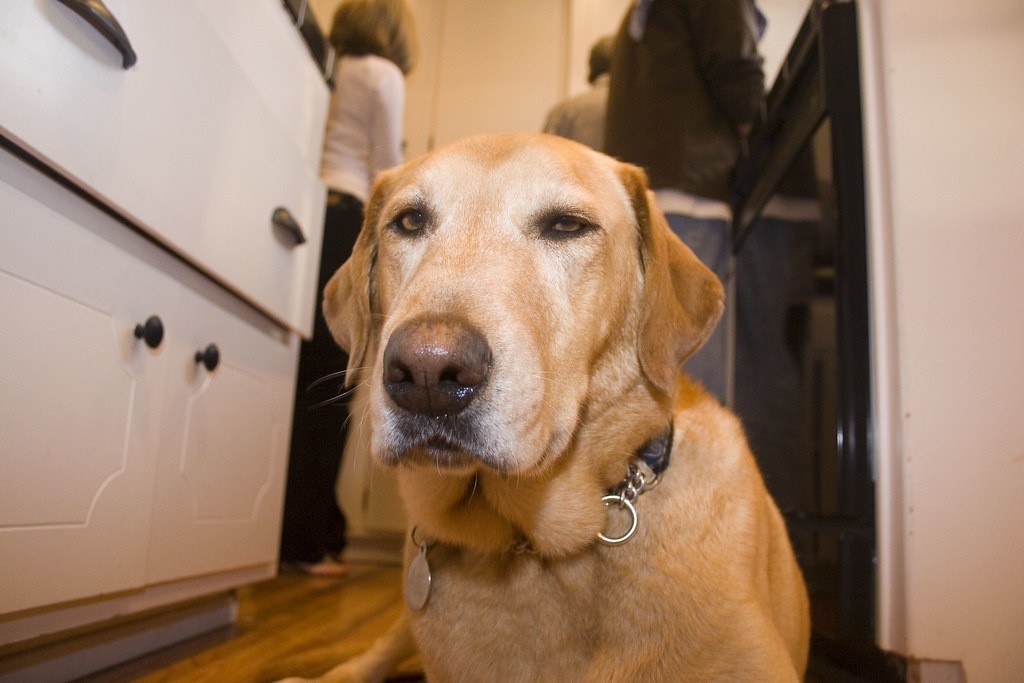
[0,0,335,683]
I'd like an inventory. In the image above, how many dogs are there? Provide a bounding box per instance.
[277,132,813,683]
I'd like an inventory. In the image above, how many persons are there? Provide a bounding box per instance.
[279,0,419,572]
[541,35,619,154]
[605,0,771,473]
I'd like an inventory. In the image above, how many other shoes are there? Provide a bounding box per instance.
[276,550,350,576]
